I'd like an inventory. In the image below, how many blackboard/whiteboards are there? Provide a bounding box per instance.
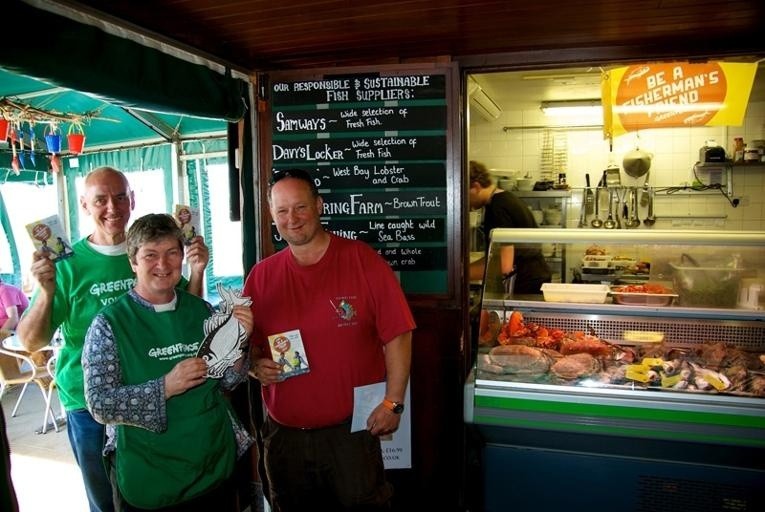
[253,62,455,300]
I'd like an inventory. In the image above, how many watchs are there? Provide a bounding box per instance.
[381,398,404,415]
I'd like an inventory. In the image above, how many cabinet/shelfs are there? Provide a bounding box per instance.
[463,227,765,512]
[507,191,571,285]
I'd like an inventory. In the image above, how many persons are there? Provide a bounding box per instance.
[233,173,415,512]
[277,352,293,374]
[0,279,31,331]
[39,238,57,258]
[54,236,72,256]
[81,213,255,511]
[291,351,308,369]
[179,221,186,242]
[15,168,211,511]
[189,224,197,242]
[468,160,551,295]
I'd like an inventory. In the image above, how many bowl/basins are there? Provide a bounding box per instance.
[530,209,561,225]
[498,177,533,192]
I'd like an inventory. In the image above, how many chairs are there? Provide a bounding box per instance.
[43,356,66,434]
[0,331,56,432]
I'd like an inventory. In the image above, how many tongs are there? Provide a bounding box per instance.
[679,253,700,268]
[586,326,667,364]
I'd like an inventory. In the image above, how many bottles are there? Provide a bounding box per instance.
[733,137,745,165]
[744,147,759,164]
[558,173,566,185]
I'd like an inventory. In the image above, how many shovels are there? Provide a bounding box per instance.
[10,127,38,176]
[585,173,594,216]
[49,130,60,174]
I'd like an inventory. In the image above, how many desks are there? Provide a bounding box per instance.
[2,335,54,351]
